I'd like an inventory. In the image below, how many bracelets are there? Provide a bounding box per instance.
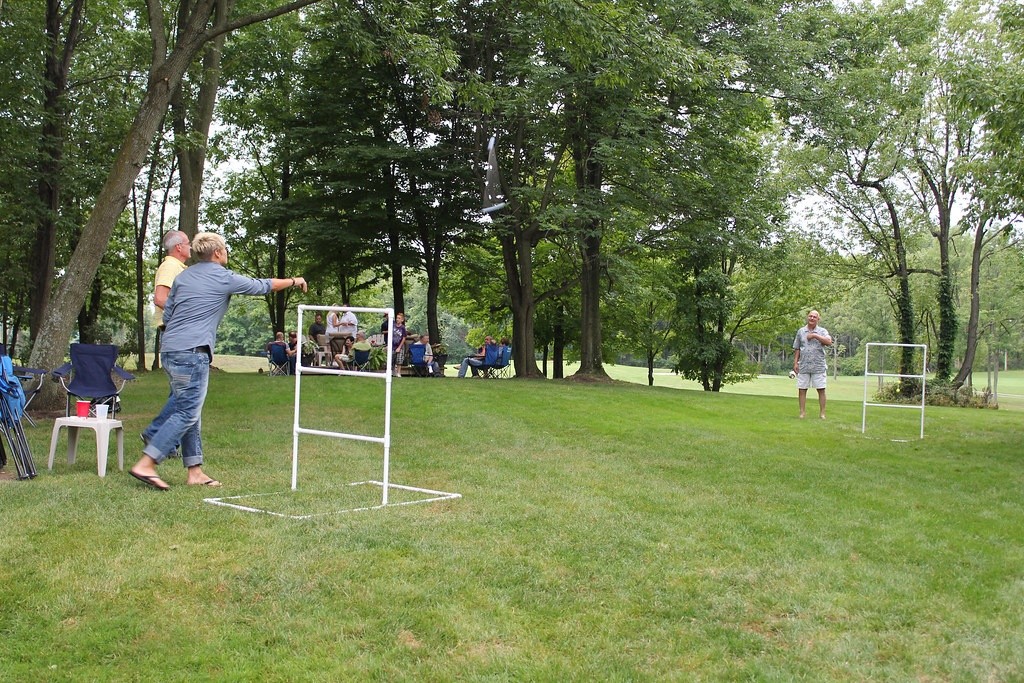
[289,277,296,287]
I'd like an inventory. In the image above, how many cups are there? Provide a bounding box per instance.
[77,401,91,419]
[95,404,109,420]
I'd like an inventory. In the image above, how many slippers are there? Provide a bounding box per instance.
[198,477,221,488]
[126,470,170,492]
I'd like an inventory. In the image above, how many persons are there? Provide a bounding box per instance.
[141,230,193,459]
[452,336,509,378]
[309,312,326,352]
[325,303,347,332]
[287,331,306,375]
[335,330,371,371]
[405,331,418,342]
[381,312,407,377]
[339,303,357,343]
[414,334,446,378]
[128,232,307,489]
[342,336,357,370]
[793,310,831,420]
[270,332,297,375]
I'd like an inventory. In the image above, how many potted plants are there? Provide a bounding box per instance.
[431,344,448,366]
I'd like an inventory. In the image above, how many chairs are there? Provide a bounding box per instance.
[266,333,372,377]
[52,343,135,419]
[467,342,512,381]
[0,343,49,479]
[407,345,438,378]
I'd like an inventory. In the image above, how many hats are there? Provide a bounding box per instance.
[382,313,387,317]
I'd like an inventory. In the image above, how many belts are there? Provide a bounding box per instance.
[190,344,209,354]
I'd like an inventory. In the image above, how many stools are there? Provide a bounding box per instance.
[47,415,123,477]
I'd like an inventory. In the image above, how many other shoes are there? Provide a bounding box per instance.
[453,365,461,369]
[392,368,397,376]
[396,374,402,378]
